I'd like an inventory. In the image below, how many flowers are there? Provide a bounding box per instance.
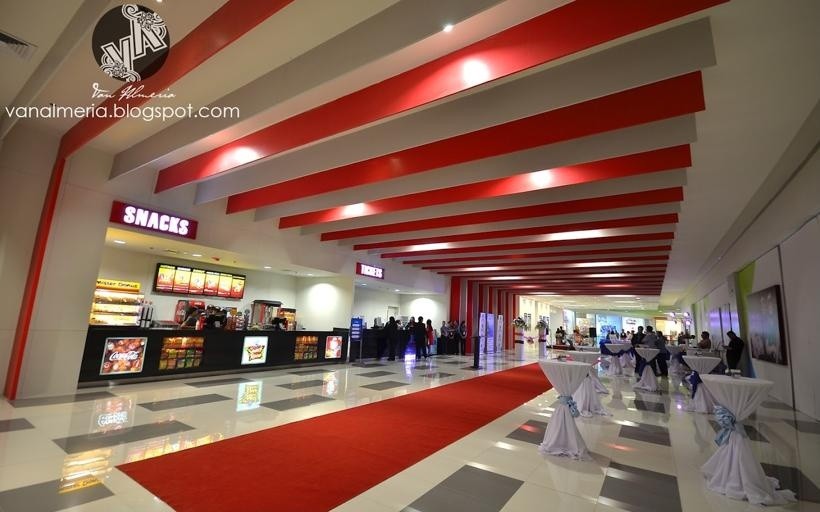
[511,316,528,330]
[535,319,548,329]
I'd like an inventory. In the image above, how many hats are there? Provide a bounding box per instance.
[207,304,220,311]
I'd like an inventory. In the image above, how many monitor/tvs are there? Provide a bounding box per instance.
[271,317,286,330]
[206,314,225,329]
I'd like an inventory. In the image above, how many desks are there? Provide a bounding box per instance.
[538,340,798,506]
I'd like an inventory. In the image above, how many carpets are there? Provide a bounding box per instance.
[117,360,555,512]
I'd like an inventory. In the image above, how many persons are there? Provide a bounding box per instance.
[696,330,712,349]
[220,308,227,324]
[384,316,400,361]
[426,319,435,357]
[575,327,583,345]
[180,304,198,329]
[559,327,566,338]
[447,320,460,355]
[447,320,454,355]
[441,320,449,355]
[720,331,745,368]
[413,316,429,359]
[458,320,467,355]
[207,304,214,312]
[605,324,671,377]
[405,316,417,329]
[215,305,221,313]
[556,328,560,338]
